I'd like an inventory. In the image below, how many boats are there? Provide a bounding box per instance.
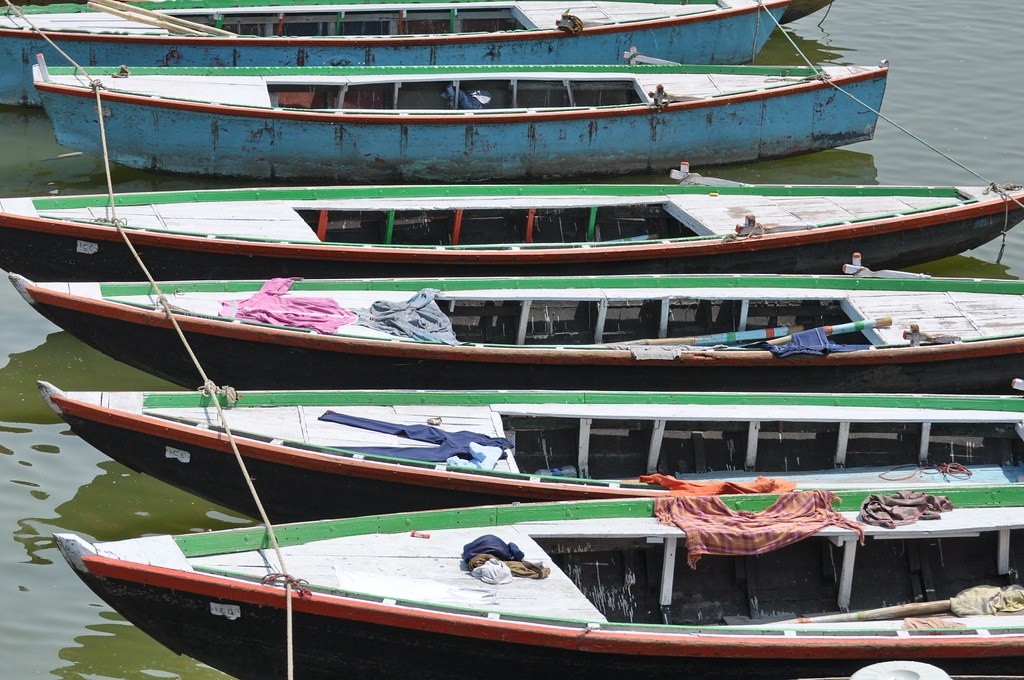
[0,2,789,107]
[33,64,887,182]
[3,184,1022,679]
